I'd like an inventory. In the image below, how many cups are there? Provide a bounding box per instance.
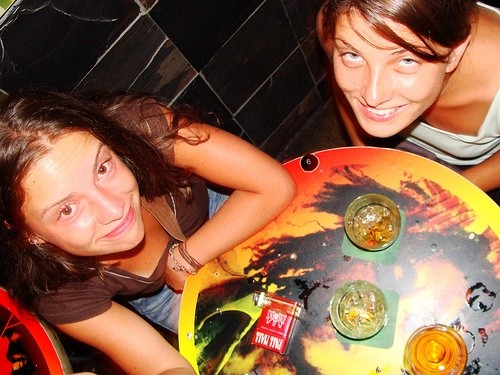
[344,194,401,251]
[329,279,388,339]
[403,323,468,375]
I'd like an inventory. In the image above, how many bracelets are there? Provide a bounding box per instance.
[168,242,203,275]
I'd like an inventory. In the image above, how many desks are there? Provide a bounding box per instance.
[179,146,500,375]
[0,286,74,375]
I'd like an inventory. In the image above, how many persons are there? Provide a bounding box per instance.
[316,0,500,192]
[0,88,298,375]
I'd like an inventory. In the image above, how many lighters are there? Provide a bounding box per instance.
[253,292,298,316]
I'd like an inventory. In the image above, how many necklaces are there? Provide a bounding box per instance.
[440,76,452,96]
